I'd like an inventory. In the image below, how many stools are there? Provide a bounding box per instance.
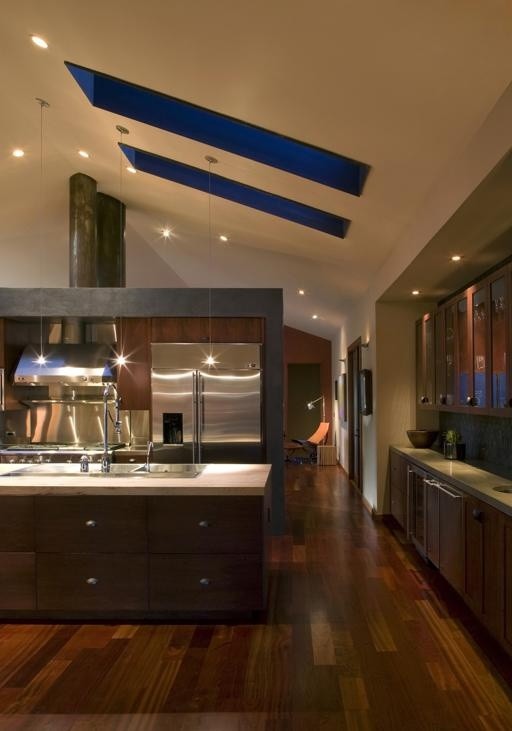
[316,445,337,466]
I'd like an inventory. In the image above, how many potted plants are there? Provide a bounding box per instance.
[443,428,468,461]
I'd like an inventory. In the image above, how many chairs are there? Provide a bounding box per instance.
[285,421,329,464]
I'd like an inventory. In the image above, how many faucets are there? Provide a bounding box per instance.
[100,383,122,472]
[146,441,154,471]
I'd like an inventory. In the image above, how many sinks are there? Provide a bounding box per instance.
[128,464,208,479]
[493,485,512,494]
[0,463,146,475]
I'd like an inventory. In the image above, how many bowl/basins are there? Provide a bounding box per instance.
[406,427,440,449]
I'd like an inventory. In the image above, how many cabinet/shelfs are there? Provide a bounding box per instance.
[417,264,512,416]
[0,488,147,622]
[151,317,265,343]
[146,477,274,622]
[386,443,512,663]
[116,318,151,411]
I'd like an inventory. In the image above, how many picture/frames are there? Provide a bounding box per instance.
[358,369,372,416]
[337,374,346,423]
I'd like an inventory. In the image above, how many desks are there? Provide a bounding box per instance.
[283,441,302,463]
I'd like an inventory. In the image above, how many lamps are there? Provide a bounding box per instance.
[115,124,129,365]
[306,395,326,424]
[204,155,218,364]
[34,97,50,366]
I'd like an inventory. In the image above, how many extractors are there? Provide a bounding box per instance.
[12,319,116,386]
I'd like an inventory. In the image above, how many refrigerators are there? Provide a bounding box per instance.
[149,340,264,463]
